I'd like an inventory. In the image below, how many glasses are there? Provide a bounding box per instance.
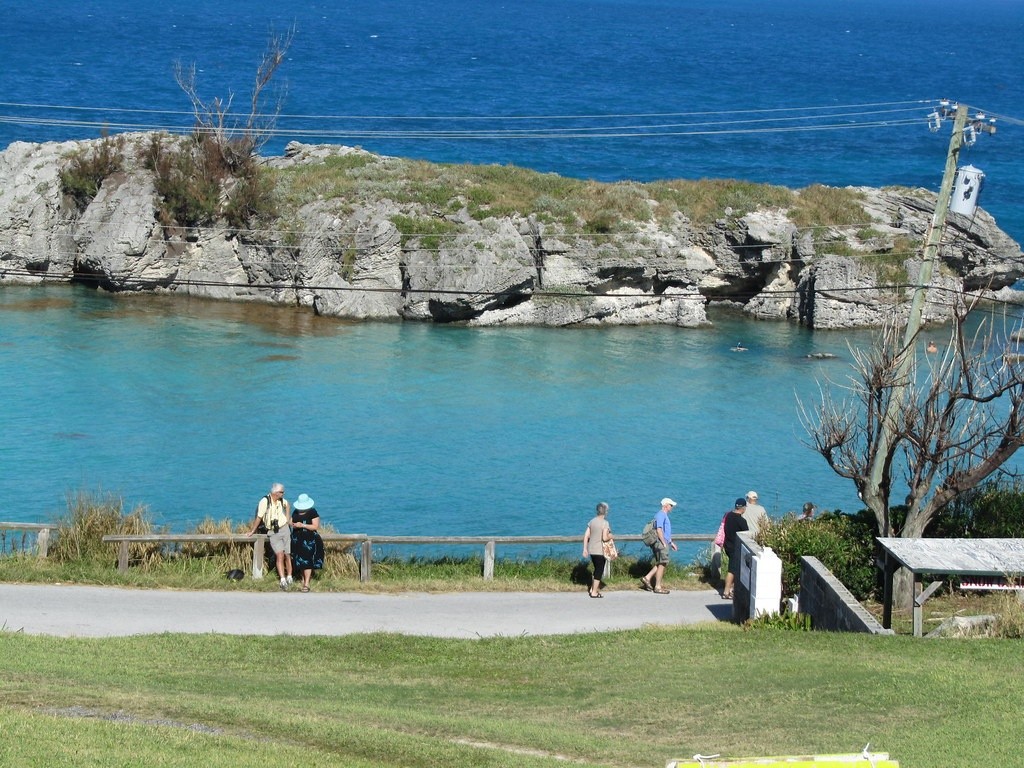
[279,492,285,494]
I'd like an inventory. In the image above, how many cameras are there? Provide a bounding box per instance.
[271,519,279,533]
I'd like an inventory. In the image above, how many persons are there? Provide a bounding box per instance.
[740,490,769,541]
[246,483,293,590]
[905,495,912,508]
[796,501,818,524]
[640,497,679,594]
[720,497,749,600]
[582,502,612,598]
[289,492,325,593]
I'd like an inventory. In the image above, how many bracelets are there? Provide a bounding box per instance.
[250,531,254,533]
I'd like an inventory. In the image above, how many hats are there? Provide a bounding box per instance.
[660,498,677,506]
[803,502,817,512]
[735,498,747,507]
[293,494,314,510]
[745,491,758,499]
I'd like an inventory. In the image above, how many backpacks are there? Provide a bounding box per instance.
[642,519,659,547]
[255,495,283,533]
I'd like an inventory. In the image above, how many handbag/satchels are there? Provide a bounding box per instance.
[713,518,725,547]
[603,539,619,562]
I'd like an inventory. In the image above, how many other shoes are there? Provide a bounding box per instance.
[301,583,312,593]
[280,578,293,591]
[589,588,604,598]
[640,577,670,594]
[722,590,734,599]
